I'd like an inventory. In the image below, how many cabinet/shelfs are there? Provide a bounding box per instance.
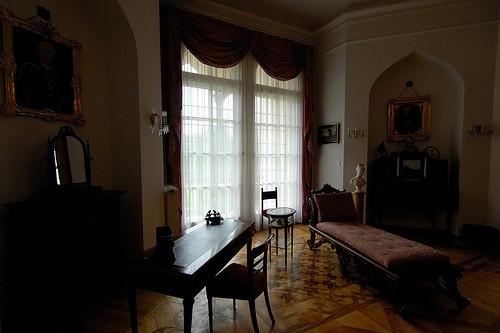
[0,188,128,333]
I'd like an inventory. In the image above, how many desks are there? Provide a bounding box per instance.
[130,219,255,333]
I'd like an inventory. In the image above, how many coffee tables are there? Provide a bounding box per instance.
[266,207,296,266]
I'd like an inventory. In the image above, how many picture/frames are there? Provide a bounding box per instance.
[386,96,431,142]
[0,7,86,126]
[425,146,441,159]
[316,123,340,146]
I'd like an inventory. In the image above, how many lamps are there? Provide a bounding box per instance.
[152,110,169,135]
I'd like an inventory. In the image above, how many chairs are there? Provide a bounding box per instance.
[260,186,278,232]
[205,233,275,333]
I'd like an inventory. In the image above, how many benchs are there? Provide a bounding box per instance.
[306,184,472,322]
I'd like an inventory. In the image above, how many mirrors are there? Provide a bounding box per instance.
[45,126,93,185]
